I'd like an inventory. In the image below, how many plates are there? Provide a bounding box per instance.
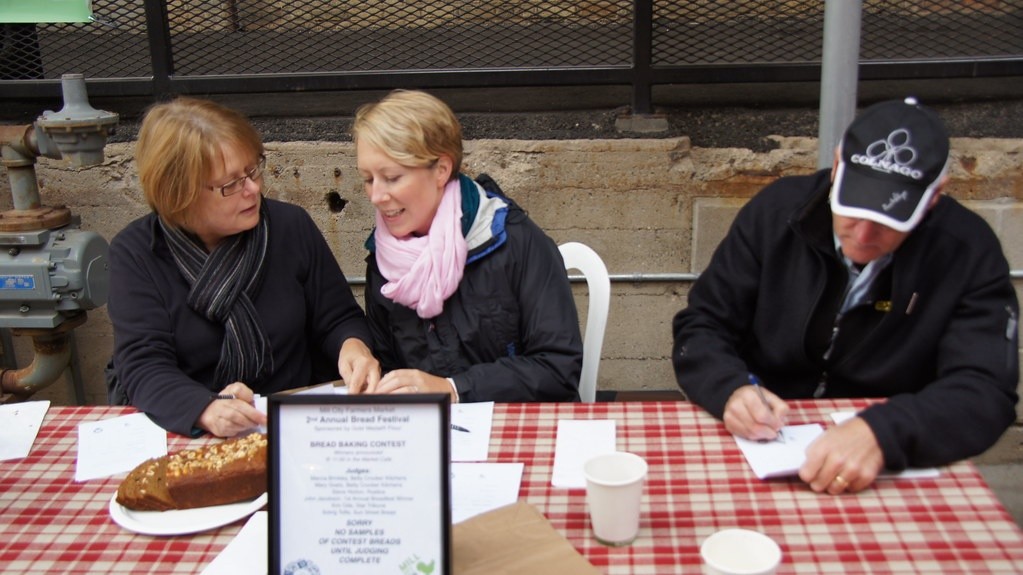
[109,491,272,536]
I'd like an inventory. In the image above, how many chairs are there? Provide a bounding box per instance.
[556,240,612,401]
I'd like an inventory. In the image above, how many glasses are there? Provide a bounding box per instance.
[201,155,267,197]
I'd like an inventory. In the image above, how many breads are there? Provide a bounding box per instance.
[117,432,268,512]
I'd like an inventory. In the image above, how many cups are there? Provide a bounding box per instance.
[584,450,649,547]
[700,528,782,574]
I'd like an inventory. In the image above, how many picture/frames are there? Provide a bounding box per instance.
[265,392,451,575]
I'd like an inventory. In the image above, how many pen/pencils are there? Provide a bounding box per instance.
[209,394,237,399]
[450,423,470,432]
[747,372,785,437]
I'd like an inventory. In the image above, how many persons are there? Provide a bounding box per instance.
[106,97,383,441]
[350,88,585,405]
[669,96,1022,495]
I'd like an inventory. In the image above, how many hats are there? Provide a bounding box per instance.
[830,98,951,234]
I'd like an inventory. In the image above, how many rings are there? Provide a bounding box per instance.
[412,385,420,395]
[835,474,850,490]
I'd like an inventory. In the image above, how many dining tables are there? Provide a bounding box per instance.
[0,395,1022,574]
